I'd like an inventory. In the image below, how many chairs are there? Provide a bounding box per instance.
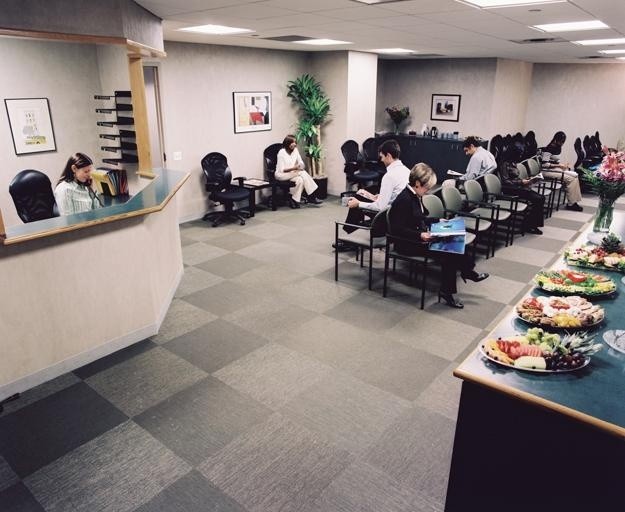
[511,156,567,217]
[10,169,61,223]
[490,131,537,166]
[341,138,386,195]
[573,131,618,195]
[335,174,530,309]
[263,143,307,211]
[202,152,251,227]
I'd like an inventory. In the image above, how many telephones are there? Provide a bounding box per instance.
[83,178,93,186]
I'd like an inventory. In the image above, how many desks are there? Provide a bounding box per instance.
[230,178,278,217]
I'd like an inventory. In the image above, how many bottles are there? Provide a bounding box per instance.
[453,132,458,139]
[422,123,428,135]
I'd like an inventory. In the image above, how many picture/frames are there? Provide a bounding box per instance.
[429,93,461,122]
[4,97,57,155]
[232,91,272,133]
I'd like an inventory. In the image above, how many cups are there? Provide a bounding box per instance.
[442,133,453,139]
[342,197,352,207]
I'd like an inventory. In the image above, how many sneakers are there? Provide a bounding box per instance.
[567,202,583,211]
[523,225,542,234]
[333,242,358,250]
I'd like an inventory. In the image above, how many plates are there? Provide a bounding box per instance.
[532,268,618,297]
[479,338,591,373]
[514,296,606,329]
[562,248,624,272]
[603,329,625,355]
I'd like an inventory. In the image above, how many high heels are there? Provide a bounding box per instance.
[460,270,489,284]
[438,290,464,308]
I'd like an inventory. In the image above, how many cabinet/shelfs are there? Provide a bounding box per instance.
[376,132,488,184]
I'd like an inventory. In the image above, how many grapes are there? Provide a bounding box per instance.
[591,247,608,257]
[546,352,583,369]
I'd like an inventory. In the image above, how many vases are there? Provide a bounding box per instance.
[593,195,614,233]
[393,123,399,135]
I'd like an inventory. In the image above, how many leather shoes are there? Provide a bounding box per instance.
[289,198,300,208]
[306,196,323,204]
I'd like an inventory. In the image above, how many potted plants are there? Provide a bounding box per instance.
[286,71,333,198]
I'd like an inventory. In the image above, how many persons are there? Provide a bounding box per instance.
[389,162,489,309]
[53,153,98,216]
[446,137,499,194]
[500,140,546,236]
[273,136,323,209]
[330,139,411,250]
[542,132,583,211]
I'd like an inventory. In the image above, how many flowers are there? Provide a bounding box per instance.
[385,105,410,123]
[581,145,625,197]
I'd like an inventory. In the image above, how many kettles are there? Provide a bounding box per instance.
[431,127,437,137]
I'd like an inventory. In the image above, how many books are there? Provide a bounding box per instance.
[242,179,269,187]
[425,217,465,255]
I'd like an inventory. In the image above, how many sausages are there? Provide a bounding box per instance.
[497,340,542,360]
